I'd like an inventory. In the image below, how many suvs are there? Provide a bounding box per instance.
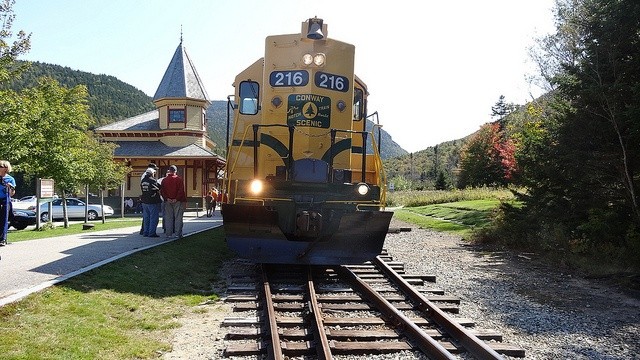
[11,193,58,229]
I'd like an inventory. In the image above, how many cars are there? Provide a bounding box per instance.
[25,198,114,222]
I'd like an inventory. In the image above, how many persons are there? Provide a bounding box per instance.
[140,163,159,235]
[124,193,142,214]
[156,169,175,233]
[205,184,228,217]
[0,161,16,247]
[160,164,185,238]
[140,168,163,237]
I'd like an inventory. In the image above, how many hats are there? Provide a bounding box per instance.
[170,165,177,171]
[146,168,156,174]
[147,163,159,170]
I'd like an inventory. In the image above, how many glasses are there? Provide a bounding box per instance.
[0,166,7,169]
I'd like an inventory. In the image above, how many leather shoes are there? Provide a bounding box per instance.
[143,234,148,236]
[149,234,159,237]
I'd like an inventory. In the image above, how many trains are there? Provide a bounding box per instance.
[221,16,394,265]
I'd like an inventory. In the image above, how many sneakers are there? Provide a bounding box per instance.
[1,240,8,246]
[165,235,171,237]
[175,235,183,238]
